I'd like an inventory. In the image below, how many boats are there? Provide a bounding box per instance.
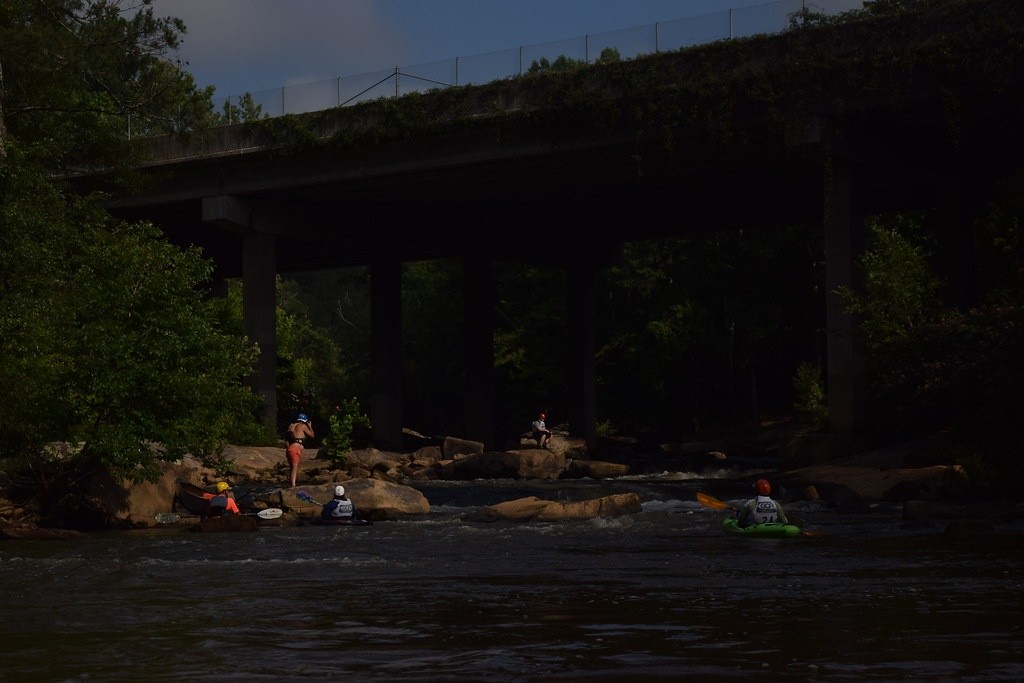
[193,510,259,533]
[722,517,801,539]
[296,514,374,527]
[175,478,240,515]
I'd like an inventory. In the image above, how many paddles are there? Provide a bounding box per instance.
[696,492,811,536]
[153,508,283,524]
[295,492,323,506]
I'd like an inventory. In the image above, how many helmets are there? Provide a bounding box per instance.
[335,486,345,496]
[756,479,771,496]
[297,413,307,423]
[539,413,546,419]
[216,481,229,493]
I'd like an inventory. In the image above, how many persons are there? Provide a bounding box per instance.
[530,414,555,451]
[286,414,315,487]
[205,482,240,517]
[735,480,789,527]
[321,485,356,521]
